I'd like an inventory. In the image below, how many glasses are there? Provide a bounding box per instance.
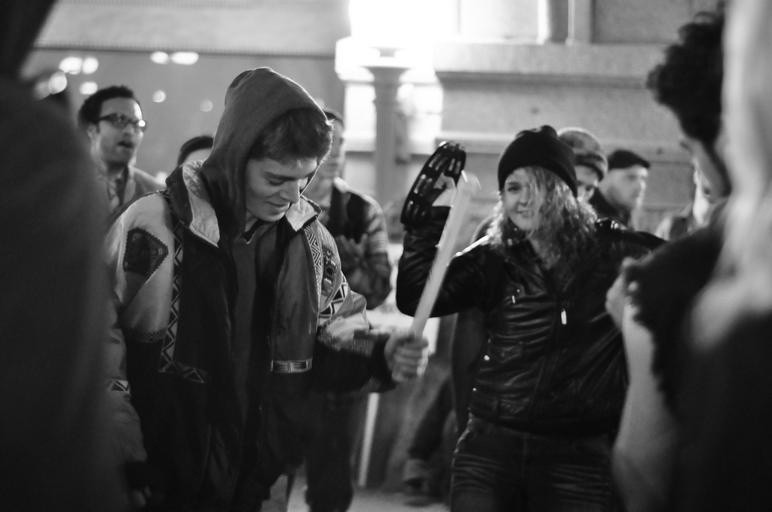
[88,113,146,132]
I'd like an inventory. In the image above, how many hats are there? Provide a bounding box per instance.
[498,126,578,197]
[557,129,609,181]
[607,149,648,170]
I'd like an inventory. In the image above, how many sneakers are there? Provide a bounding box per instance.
[402,460,433,499]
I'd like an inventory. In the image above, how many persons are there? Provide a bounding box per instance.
[0,2,150,511]
[394,126,707,511]
[602,2,771,512]
[73,85,393,510]
[101,66,430,511]
